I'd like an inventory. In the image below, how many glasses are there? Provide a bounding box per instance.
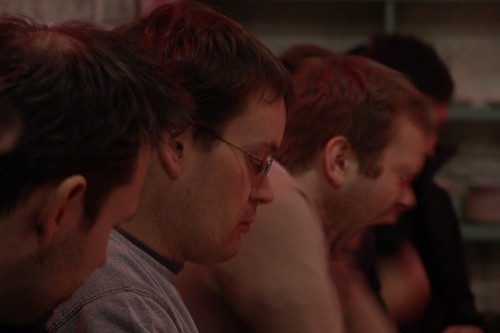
[208,130,273,183]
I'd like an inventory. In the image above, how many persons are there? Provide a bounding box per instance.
[46,3,294,333]
[177,34,483,333]
[0,27,197,333]
